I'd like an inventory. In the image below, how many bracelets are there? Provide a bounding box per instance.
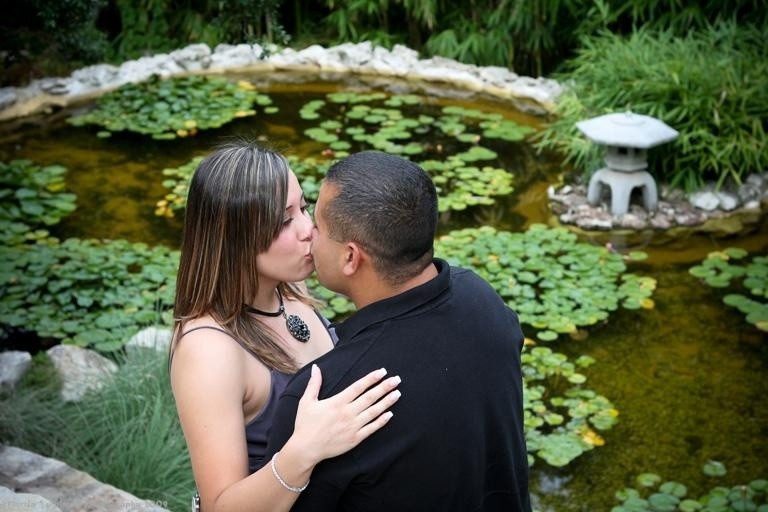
[271,452,311,493]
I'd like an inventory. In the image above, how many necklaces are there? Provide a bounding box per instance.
[242,283,311,342]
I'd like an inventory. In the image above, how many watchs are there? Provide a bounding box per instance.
[191,490,200,512]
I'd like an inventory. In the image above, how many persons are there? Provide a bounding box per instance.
[166,142,402,512]
[191,150,534,512]
